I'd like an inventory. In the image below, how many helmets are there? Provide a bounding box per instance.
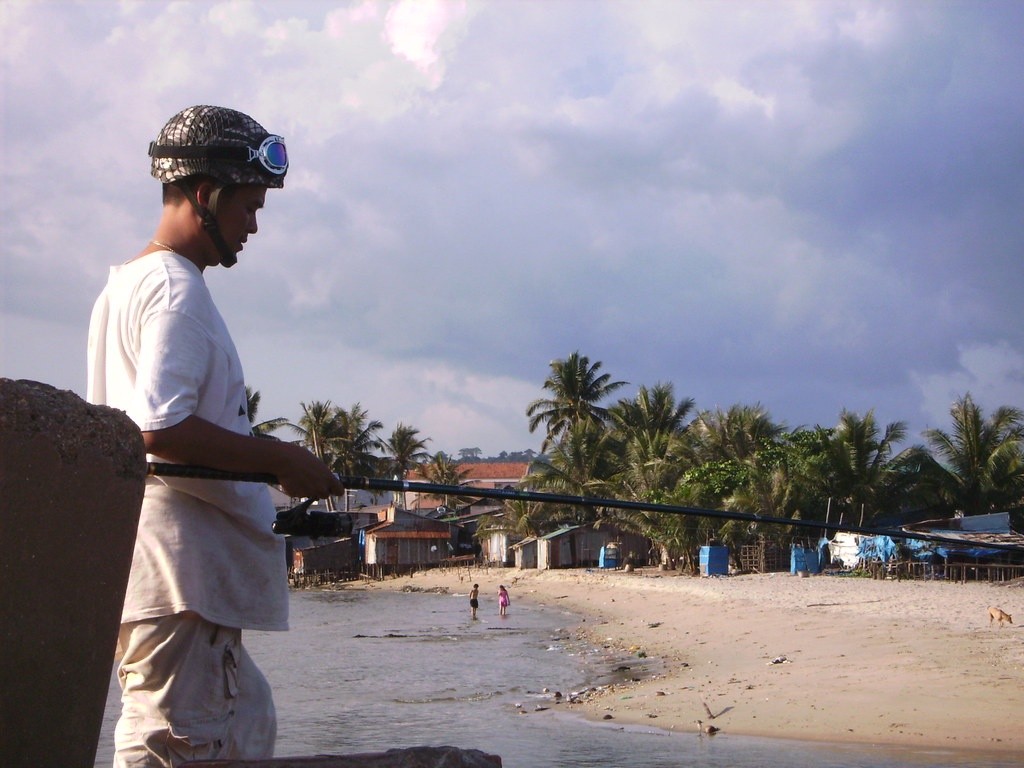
[151,105,285,188]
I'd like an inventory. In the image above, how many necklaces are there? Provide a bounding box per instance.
[150,240,176,253]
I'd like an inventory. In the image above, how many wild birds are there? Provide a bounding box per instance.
[702,702,735,719]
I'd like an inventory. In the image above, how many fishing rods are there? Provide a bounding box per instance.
[145,461,1024,554]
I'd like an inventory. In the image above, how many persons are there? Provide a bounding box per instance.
[498,585,510,616]
[86,106,347,768]
[469,584,479,620]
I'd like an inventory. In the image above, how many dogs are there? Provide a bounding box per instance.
[986,605,1014,628]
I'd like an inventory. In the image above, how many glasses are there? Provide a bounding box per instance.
[148,135,289,177]
